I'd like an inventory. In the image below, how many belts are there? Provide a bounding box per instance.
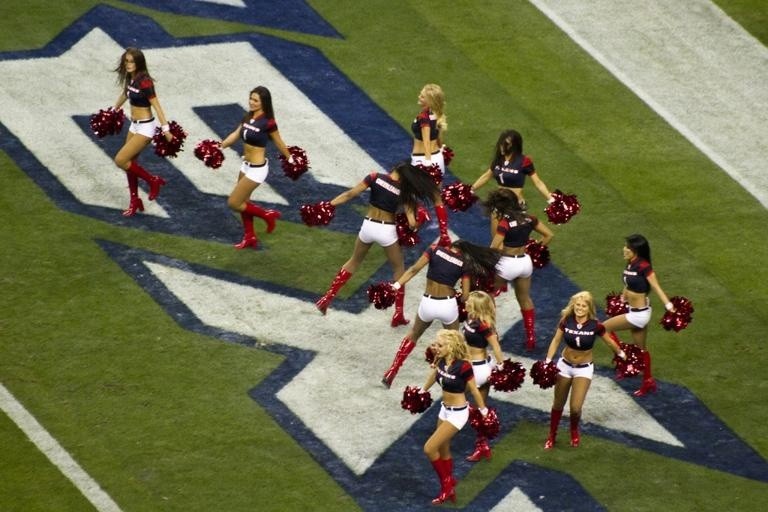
[245,160,267,167]
[503,254,525,258]
[447,406,467,411]
[472,356,491,365]
[412,150,440,156]
[365,217,395,225]
[631,308,648,312]
[562,359,593,368]
[423,294,454,300]
[133,117,154,123]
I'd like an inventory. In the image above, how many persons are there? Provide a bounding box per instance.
[90,46,187,218]
[487,187,555,352]
[397,328,501,507]
[595,233,695,397]
[362,237,504,390]
[299,163,441,329]
[191,86,312,251]
[399,83,456,248]
[444,130,582,245]
[530,290,646,450]
[455,290,526,463]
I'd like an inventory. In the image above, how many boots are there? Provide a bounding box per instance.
[234,211,257,249]
[241,201,281,233]
[545,407,563,449]
[391,285,409,327]
[435,205,451,246]
[570,413,580,447]
[633,351,657,397]
[416,204,432,226]
[430,454,458,505]
[125,161,166,201]
[466,440,491,462]
[122,169,144,217]
[521,309,537,352]
[383,336,416,384]
[316,267,353,315]
